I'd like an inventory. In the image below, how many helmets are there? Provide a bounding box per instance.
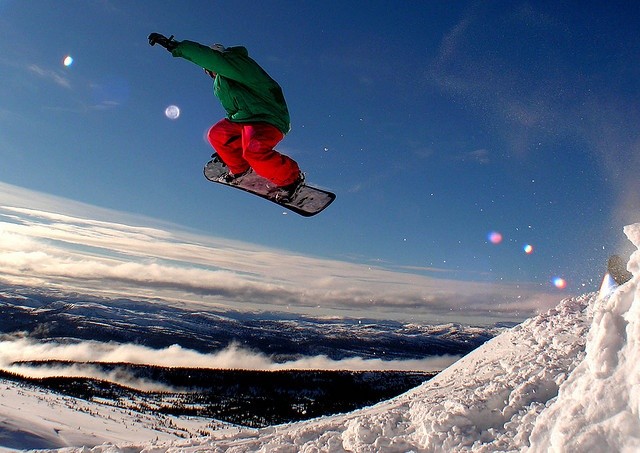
[202,43,225,74]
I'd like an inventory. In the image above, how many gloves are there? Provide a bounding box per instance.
[148,33,174,48]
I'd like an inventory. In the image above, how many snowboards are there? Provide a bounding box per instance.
[204,158,336,217]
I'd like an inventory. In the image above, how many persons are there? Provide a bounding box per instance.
[147,33,301,190]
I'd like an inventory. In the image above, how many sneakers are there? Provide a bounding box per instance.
[275,170,306,203]
[219,166,253,184]
